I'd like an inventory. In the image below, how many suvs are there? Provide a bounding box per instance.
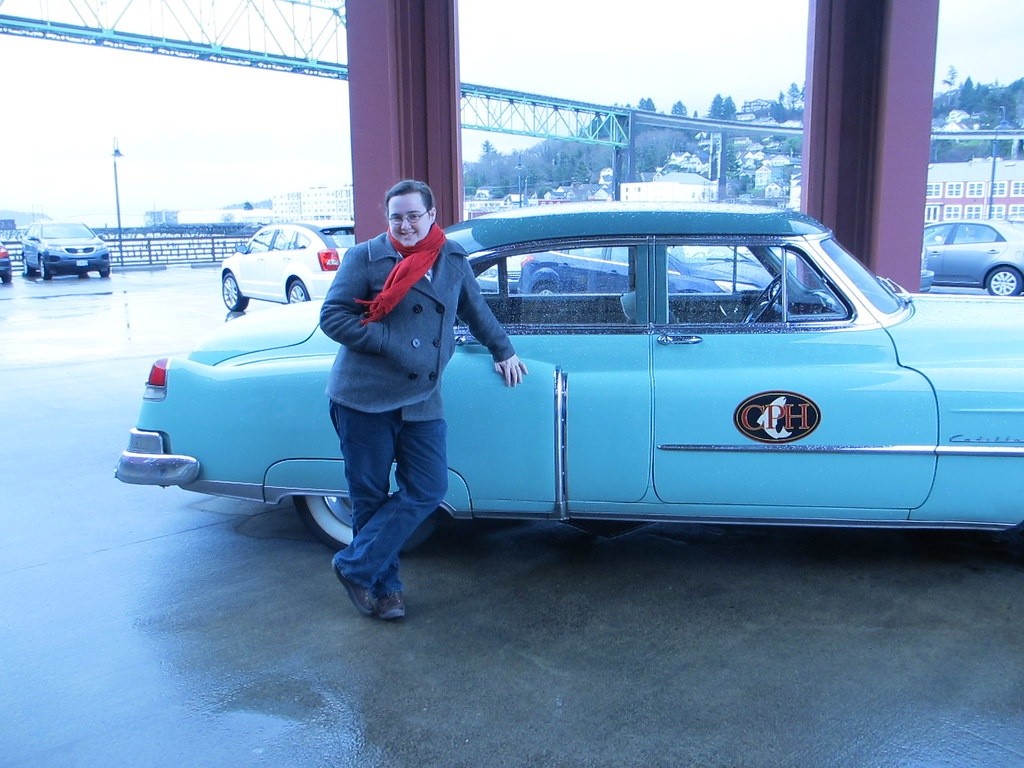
[22,221,111,281]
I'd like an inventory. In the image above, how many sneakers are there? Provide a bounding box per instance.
[374,590,405,619]
[332,558,375,615]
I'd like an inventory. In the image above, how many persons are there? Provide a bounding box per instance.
[317,179,528,618]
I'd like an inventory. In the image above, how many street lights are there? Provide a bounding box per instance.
[987,105,1013,217]
[112,137,127,267]
[515,155,526,207]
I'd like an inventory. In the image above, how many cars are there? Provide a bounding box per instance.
[921,217,1024,296]
[517,243,777,297]
[0,243,13,284]
[474,256,522,295]
[220,217,356,311]
[115,200,1024,560]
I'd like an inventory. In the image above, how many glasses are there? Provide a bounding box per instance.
[386,206,433,225]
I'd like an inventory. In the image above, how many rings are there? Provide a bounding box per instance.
[516,366,519,368]
